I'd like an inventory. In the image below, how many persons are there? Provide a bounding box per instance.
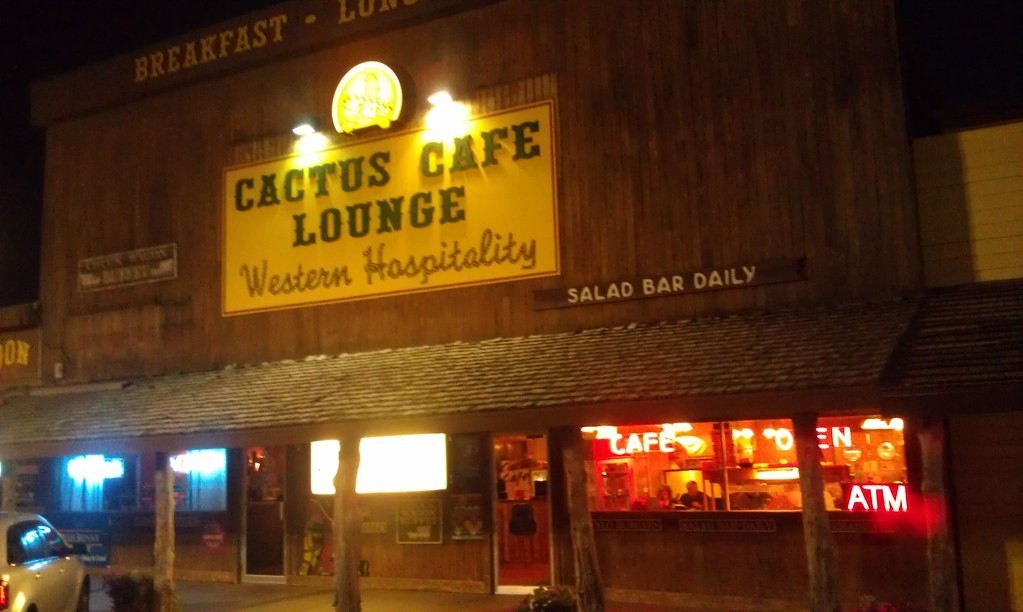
[657,485,681,509]
[509,489,540,565]
[680,481,712,509]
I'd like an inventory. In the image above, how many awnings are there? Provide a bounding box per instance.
[0,279,1022,458]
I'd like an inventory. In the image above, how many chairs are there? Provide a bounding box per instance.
[509,503,536,564]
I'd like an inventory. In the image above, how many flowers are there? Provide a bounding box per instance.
[517,584,577,612]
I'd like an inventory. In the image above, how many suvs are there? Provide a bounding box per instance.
[0,509,92,612]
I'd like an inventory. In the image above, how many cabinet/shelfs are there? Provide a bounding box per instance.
[600,463,630,510]
